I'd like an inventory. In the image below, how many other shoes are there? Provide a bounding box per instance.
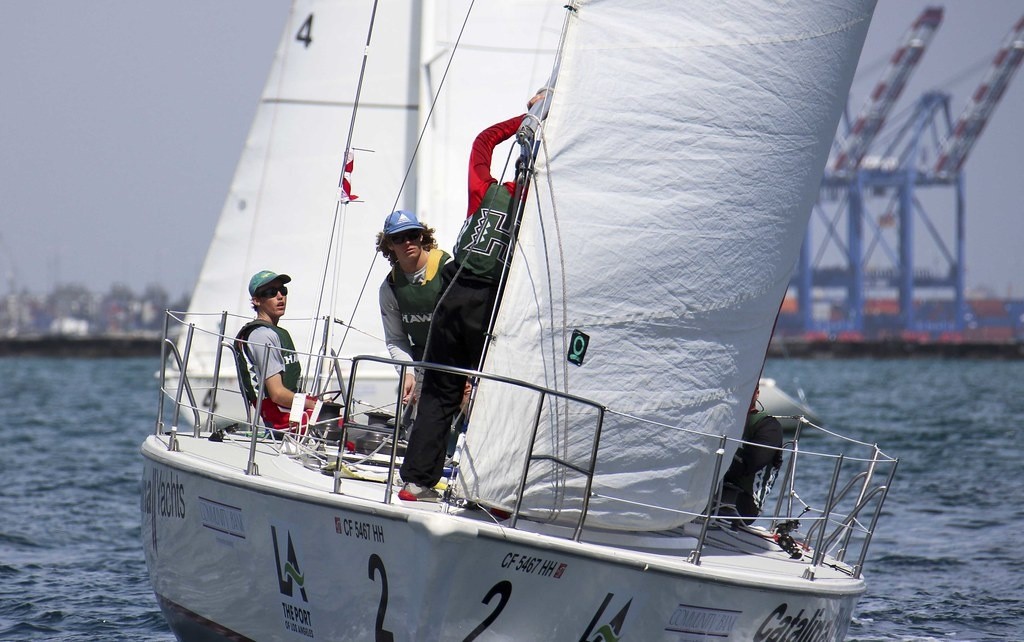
[387,418,396,425]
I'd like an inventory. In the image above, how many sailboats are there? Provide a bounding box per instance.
[139,1,899,641]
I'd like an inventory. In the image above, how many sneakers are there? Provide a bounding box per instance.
[398,482,443,501]
[490,508,510,519]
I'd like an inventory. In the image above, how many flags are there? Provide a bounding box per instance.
[337,148,358,204]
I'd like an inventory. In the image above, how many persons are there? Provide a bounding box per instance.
[710,382,784,528]
[398,94,548,520]
[376,212,474,459]
[233,270,359,452]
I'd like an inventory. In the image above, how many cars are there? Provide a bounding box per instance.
[50,313,103,335]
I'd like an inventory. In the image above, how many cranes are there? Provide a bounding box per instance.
[775,5,1024,334]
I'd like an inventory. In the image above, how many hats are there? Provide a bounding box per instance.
[249,271,291,297]
[384,210,425,234]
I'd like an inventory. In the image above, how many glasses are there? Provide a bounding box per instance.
[257,287,287,297]
[390,230,419,244]
